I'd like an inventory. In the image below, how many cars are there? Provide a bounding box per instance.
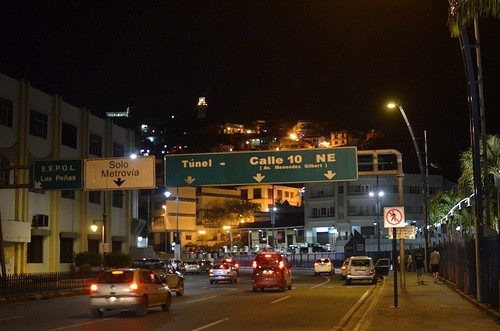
[90,268,172,318]
[198,260,212,272]
[376,257,390,276]
[209,259,240,283]
[314,258,335,276]
[342,258,350,279]
[165,257,200,275]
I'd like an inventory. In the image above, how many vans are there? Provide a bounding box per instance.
[347,256,377,285]
[253,252,293,292]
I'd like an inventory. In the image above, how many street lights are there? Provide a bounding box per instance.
[164,191,179,270]
[370,191,380,256]
[91,220,104,272]
[271,207,277,252]
[388,102,427,274]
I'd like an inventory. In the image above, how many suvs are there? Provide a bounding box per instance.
[134,257,185,296]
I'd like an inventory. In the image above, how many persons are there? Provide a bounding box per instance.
[397,252,412,272]
[414,250,425,285]
[429,247,440,283]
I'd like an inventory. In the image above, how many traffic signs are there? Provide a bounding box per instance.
[83,155,156,191]
[33,159,83,191]
[164,146,358,189]
